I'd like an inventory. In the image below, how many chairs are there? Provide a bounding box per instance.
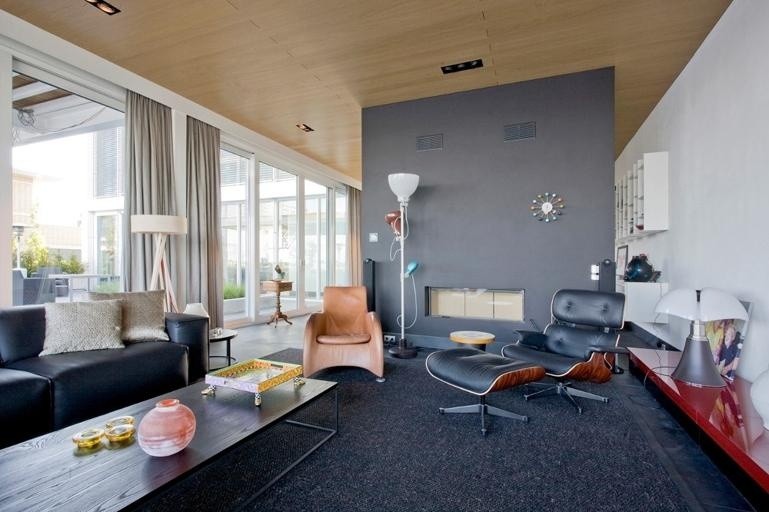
[424,284,630,439]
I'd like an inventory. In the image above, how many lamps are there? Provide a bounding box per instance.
[656,283,754,389]
[181,303,208,319]
[382,169,429,360]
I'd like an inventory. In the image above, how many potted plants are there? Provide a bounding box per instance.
[273,266,285,281]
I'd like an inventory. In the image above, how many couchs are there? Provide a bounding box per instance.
[301,282,386,383]
[0,279,222,440]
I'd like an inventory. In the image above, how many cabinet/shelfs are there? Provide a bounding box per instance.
[607,148,671,244]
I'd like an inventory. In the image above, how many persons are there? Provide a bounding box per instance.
[706,318,745,381]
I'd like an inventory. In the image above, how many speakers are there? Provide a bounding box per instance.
[599,259,616,293]
[362,258,375,311]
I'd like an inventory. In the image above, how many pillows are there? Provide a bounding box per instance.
[72,287,175,342]
[33,296,126,355]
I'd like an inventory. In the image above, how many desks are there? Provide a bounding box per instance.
[263,280,293,328]
[0,360,339,512]
[208,325,238,370]
